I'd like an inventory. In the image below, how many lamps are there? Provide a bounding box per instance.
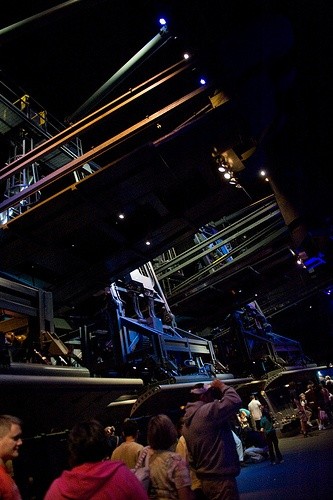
[218,161,237,185]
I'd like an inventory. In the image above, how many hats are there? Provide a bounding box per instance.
[190,383,212,394]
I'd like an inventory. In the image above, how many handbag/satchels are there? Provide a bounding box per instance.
[129,445,150,493]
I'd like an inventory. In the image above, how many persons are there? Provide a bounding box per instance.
[289,375,333,438]
[181,379,242,500]
[102,395,284,500]
[0,414,24,500]
[44,419,150,500]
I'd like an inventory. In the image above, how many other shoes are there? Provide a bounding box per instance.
[280,459,284,464]
[270,462,276,465]
